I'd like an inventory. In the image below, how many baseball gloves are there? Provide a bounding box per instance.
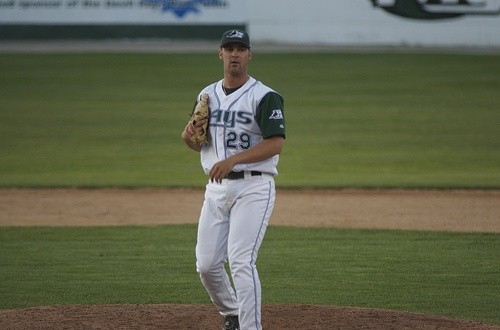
[182,93,211,152]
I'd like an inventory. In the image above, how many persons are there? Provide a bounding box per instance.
[180,30,286,330]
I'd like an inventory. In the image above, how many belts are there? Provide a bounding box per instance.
[223,170,262,179]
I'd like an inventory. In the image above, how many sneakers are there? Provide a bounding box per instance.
[224,316,239,330]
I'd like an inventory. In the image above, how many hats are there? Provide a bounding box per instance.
[220,29,250,49]
